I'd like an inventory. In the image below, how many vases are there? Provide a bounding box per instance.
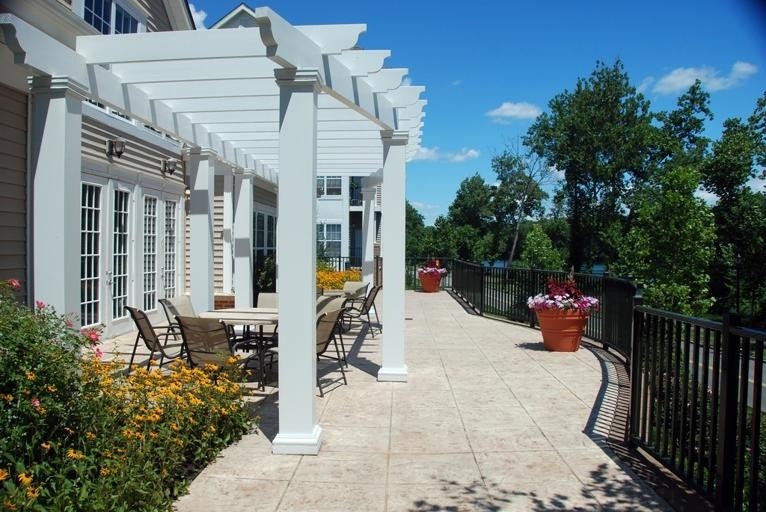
[534,305,586,352]
[419,272,442,292]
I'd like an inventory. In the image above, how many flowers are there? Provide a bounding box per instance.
[415,259,450,277]
[527,267,599,314]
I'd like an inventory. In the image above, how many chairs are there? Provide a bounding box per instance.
[125,279,382,396]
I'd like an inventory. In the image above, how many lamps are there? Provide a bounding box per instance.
[161,155,177,175]
[106,135,127,158]
